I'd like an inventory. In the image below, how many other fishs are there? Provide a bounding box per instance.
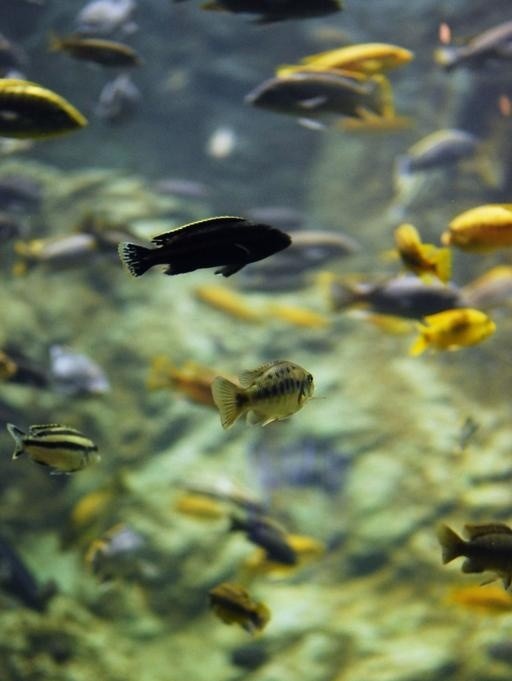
[1,1,512,636]
